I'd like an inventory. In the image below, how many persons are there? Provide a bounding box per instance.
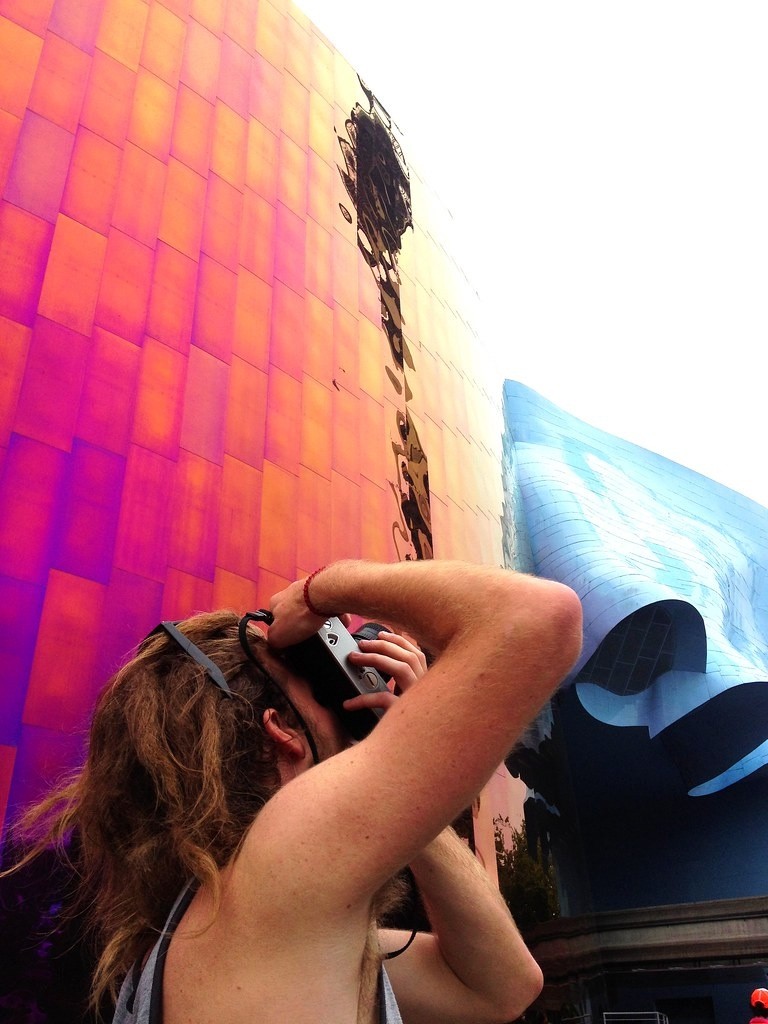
[0,559,582,1024]
[749,988,768,1024]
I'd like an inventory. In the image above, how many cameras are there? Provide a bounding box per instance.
[301,617,395,742]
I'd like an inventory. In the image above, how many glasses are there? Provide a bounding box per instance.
[143,620,234,699]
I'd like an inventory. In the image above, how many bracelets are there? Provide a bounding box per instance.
[303,566,344,617]
